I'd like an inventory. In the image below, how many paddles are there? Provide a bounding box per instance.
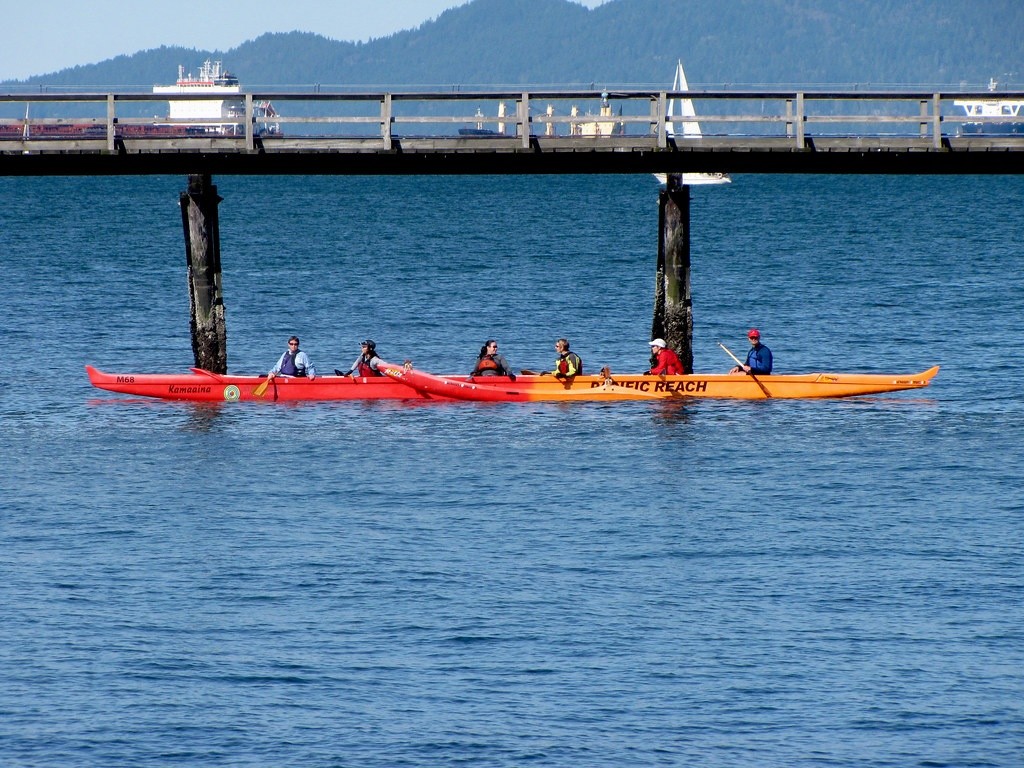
[656,372,684,400]
[600,365,611,378]
[253,369,281,397]
[334,369,355,380]
[521,369,556,376]
[716,341,771,398]
[465,373,476,380]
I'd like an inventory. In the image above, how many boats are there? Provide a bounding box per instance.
[952,77,1024,137]
[84,361,940,404]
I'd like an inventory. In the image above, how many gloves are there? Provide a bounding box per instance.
[643,371,651,375]
[556,373,566,378]
[540,371,547,376]
[509,374,516,381]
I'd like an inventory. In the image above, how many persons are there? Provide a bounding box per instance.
[268,336,316,381]
[345,340,387,377]
[470,340,516,379]
[540,339,582,377]
[643,338,684,375]
[730,330,772,375]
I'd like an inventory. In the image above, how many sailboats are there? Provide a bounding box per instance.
[653,59,731,187]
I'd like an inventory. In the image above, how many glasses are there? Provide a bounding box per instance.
[289,343,298,345]
[652,346,655,347]
[556,345,560,348]
[490,346,497,348]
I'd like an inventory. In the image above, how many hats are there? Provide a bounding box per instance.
[358,340,376,348]
[748,330,759,337]
[648,338,666,347]
